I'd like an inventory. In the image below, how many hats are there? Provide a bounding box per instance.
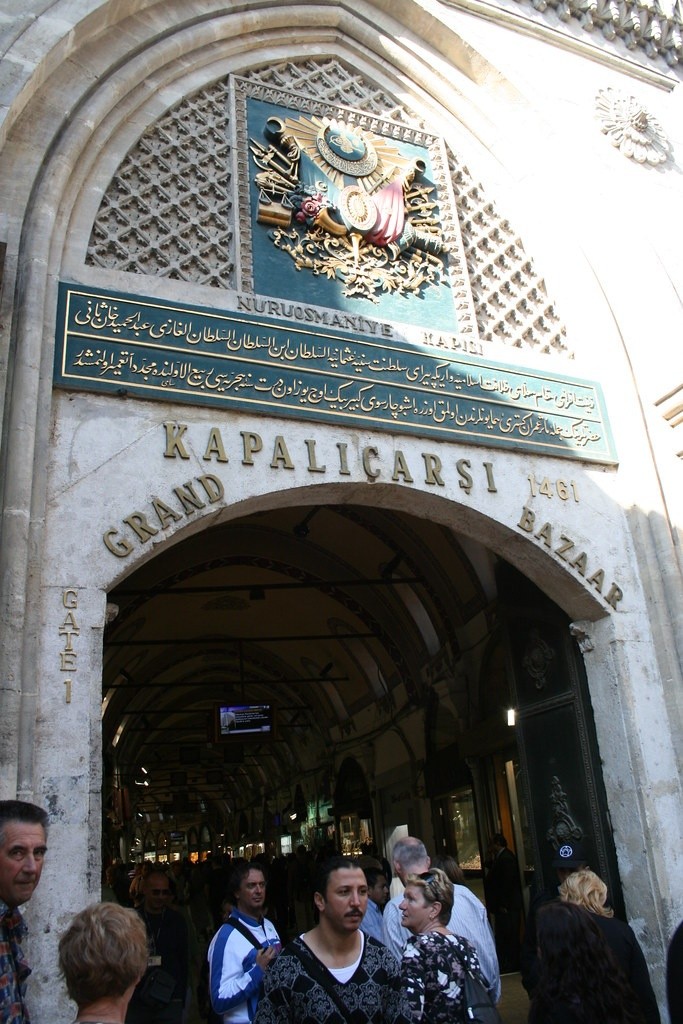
[551,842,582,869]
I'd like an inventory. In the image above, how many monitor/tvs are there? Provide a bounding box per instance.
[214,700,276,743]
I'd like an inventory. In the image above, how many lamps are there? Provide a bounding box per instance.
[144,778,151,786]
[287,809,297,820]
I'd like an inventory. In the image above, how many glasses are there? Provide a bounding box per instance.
[417,870,438,886]
[147,887,171,897]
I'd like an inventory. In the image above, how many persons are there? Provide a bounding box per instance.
[523,843,615,995]
[125,870,187,1024]
[253,858,413,1024]
[166,888,202,1024]
[557,870,661,1024]
[399,867,500,1024]
[0,800,48,1024]
[58,902,149,1024]
[485,835,531,974]
[357,843,391,886]
[206,863,281,1024]
[528,902,646,1024]
[383,837,500,1005]
[106,840,339,941]
[430,855,466,885]
[359,866,388,943]
[198,899,237,1024]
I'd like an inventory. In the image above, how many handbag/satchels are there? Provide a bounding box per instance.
[435,929,502,1024]
[135,958,175,1013]
